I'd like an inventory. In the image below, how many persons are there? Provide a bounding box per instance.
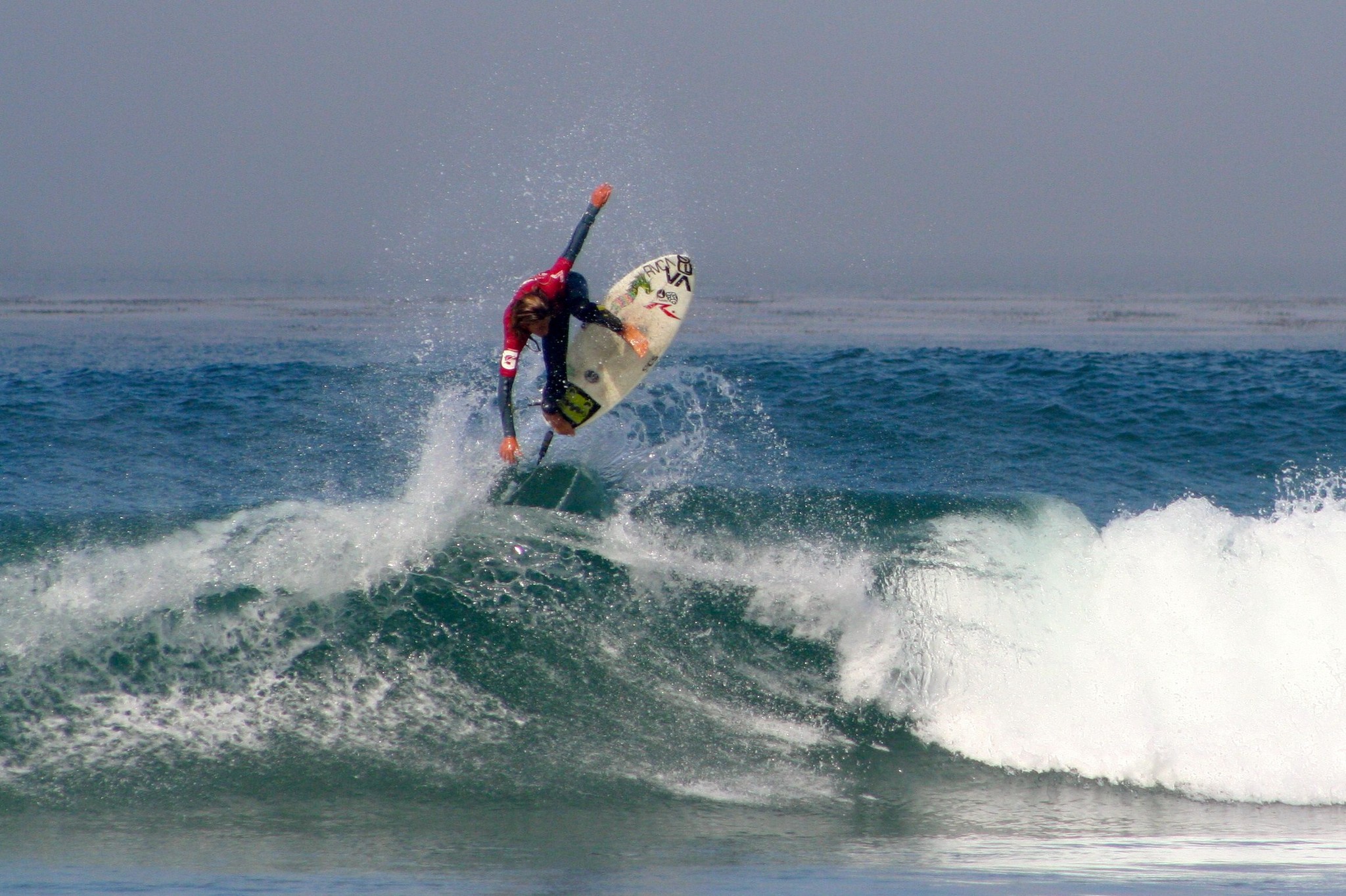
[498,181,649,463]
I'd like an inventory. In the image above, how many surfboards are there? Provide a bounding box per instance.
[543,255,695,435]
